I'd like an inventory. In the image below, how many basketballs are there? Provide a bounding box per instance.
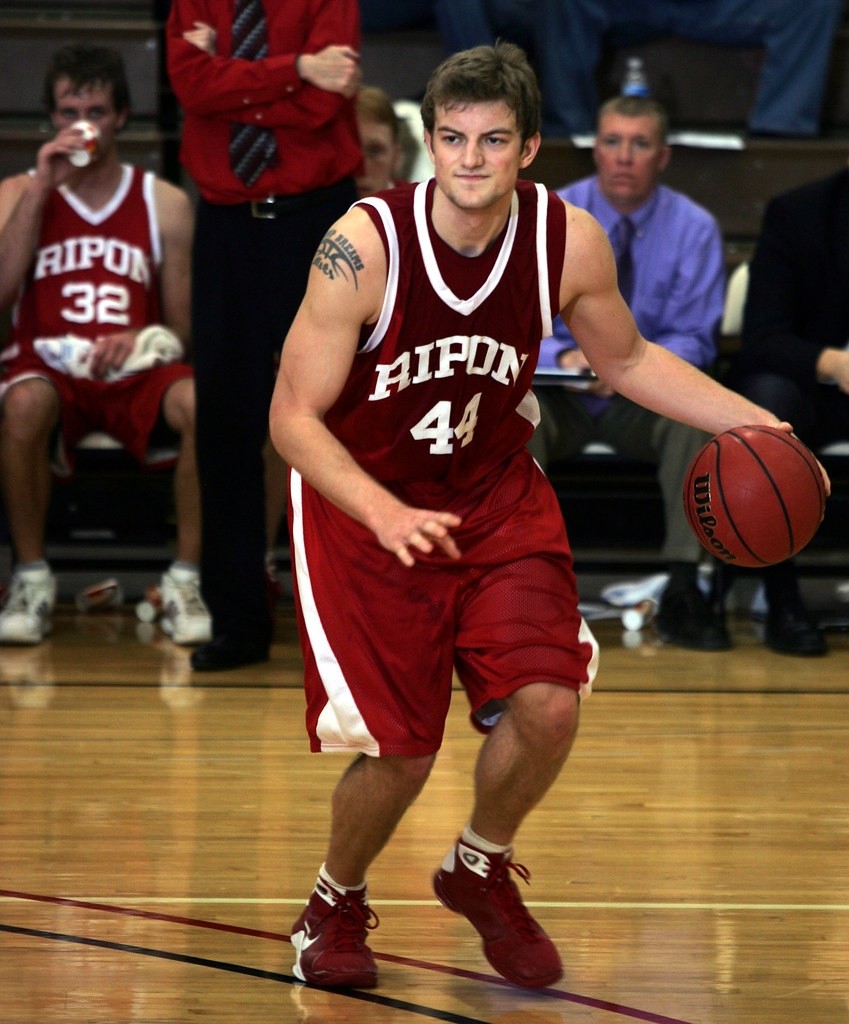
[677,420,833,571]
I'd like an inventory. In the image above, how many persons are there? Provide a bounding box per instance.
[0,44,217,647]
[165,0,363,673]
[533,94,731,654]
[270,45,832,989]
[431,0,842,142]
[738,167,849,656]
[350,83,407,200]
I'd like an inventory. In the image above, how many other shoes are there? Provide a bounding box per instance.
[193,619,271,672]
[655,585,732,651]
[766,606,828,656]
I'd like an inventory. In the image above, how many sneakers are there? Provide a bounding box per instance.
[156,559,212,644]
[432,837,564,990]
[0,573,56,643]
[290,874,380,990]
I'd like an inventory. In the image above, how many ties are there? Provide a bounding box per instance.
[611,217,636,309]
[230,0,277,186]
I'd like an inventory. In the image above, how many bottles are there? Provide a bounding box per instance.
[620,57,649,98]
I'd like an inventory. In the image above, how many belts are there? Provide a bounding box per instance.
[232,180,356,220]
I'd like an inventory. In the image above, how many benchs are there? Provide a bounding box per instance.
[0,17,849,562]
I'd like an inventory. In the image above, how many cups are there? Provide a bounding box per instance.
[68,120,102,168]
